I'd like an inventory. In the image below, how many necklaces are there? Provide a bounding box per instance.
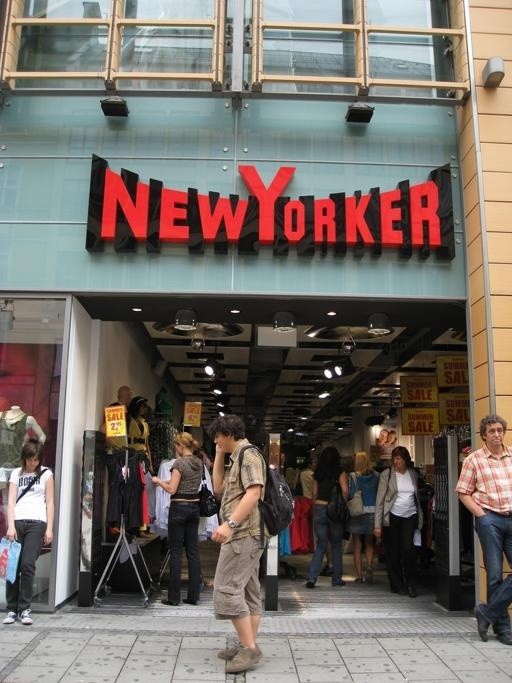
[2,411,24,420]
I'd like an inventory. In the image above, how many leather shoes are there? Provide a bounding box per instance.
[162,600,178,605]
[473,607,490,642]
[135,530,150,538]
[183,598,197,604]
[406,584,416,597]
[497,633,512,646]
[398,589,407,596]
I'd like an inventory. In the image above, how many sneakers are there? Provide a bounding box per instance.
[225,644,262,673]
[306,582,314,589]
[3,611,18,624]
[333,581,345,586]
[218,642,244,659]
[321,565,333,576]
[355,578,363,584]
[365,566,372,584]
[19,609,33,624]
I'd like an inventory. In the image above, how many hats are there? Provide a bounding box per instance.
[128,397,148,411]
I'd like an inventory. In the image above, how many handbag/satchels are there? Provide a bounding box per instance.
[326,499,349,522]
[198,483,218,517]
[1,536,21,584]
[347,489,363,518]
[294,472,303,495]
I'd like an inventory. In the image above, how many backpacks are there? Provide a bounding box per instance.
[238,445,295,537]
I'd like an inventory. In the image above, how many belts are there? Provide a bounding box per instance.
[128,437,145,445]
[21,520,44,525]
[171,500,200,505]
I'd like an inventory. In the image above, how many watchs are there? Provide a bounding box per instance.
[226,518,238,529]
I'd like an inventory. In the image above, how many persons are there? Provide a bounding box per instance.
[210,414,272,673]
[349,451,382,584]
[3,438,55,624]
[307,448,350,588]
[129,396,153,538]
[285,459,299,497]
[151,432,205,605]
[373,446,423,597]
[100,386,133,545]
[0,407,47,530]
[380,431,397,455]
[297,457,318,500]
[454,416,512,646]
[377,429,389,449]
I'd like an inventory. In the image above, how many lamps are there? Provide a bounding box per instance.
[285,327,357,433]
[100,96,129,117]
[345,101,375,123]
[272,313,296,334]
[183,334,232,418]
[367,313,391,335]
[386,398,398,420]
[365,406,384,427]
[172,309,198,331]
